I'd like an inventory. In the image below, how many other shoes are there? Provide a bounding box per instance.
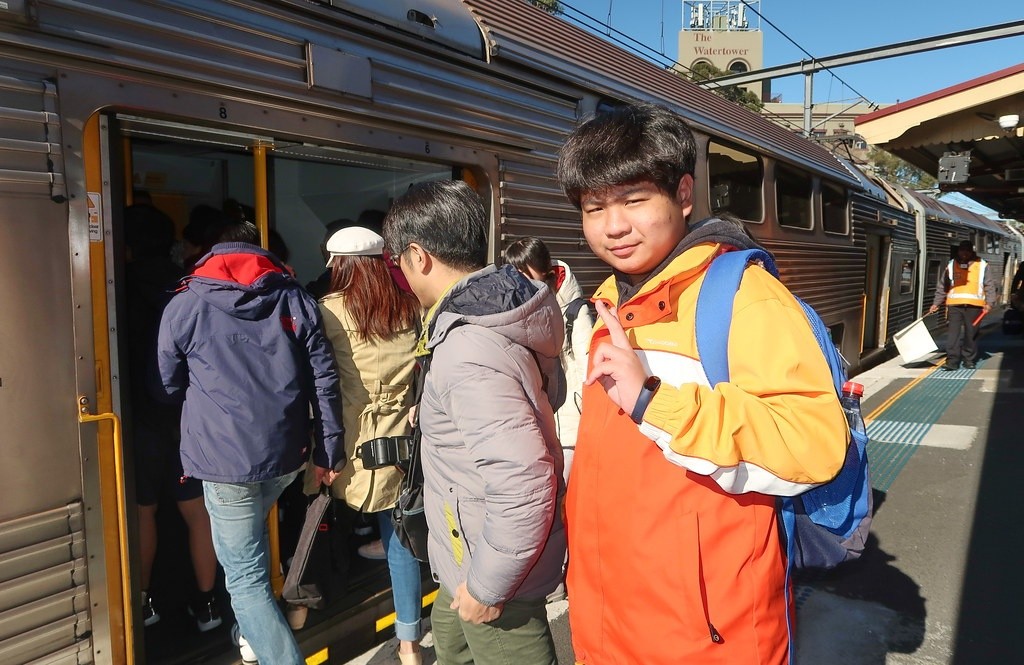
[941,363,957,370]
[142,593,160,627]
[964,362,975,369]
[186,598,222,632]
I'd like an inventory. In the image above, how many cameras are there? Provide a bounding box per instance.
[360,435,415,470]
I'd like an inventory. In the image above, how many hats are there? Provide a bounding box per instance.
[960,240,973,249]
[326,226,384,268]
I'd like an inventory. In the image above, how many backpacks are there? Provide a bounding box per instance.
[695,248,874,665]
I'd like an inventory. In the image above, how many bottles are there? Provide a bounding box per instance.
[839,382,866,436]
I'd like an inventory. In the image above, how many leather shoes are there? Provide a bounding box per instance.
[391,643,422,665]
[286,608,307,629]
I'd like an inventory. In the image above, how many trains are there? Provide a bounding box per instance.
[0,0,1024,665]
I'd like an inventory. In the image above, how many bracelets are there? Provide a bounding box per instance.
[631,376,661,424]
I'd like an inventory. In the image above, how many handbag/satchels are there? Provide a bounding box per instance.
[281,447,347,611]
[391,482,429,562]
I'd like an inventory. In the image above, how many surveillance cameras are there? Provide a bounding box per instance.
[999,115,1019,132]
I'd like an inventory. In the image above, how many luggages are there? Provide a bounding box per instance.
[1003,306,1022,335]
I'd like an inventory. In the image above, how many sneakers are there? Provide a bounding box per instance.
[358,539,386,559]
[231,623,259,665]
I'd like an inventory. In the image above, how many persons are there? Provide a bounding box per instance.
[109,201,389,630]
[930,240,997,370]
[1011,260,1024,313]
[558,102,851,665]
[304,226,430,665]
[157,197,347,665]
[503,237,595,665]
[383,177,568,665]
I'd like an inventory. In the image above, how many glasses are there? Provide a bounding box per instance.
[390,245,410,269]
